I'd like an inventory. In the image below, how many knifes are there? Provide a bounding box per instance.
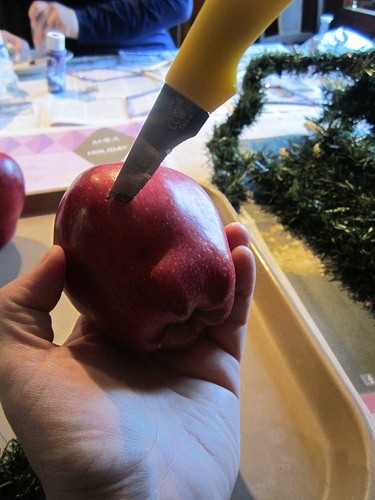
[108,0,294,203]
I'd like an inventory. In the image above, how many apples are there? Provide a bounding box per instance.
[53,161,237,358]
[0,152,25,252]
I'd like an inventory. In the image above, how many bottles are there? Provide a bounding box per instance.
[45,32,66,94]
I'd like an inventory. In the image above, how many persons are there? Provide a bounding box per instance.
[0,221,258,500]
[0,0,195,58]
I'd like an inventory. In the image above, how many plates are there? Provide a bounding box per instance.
[11,49,73,76]
